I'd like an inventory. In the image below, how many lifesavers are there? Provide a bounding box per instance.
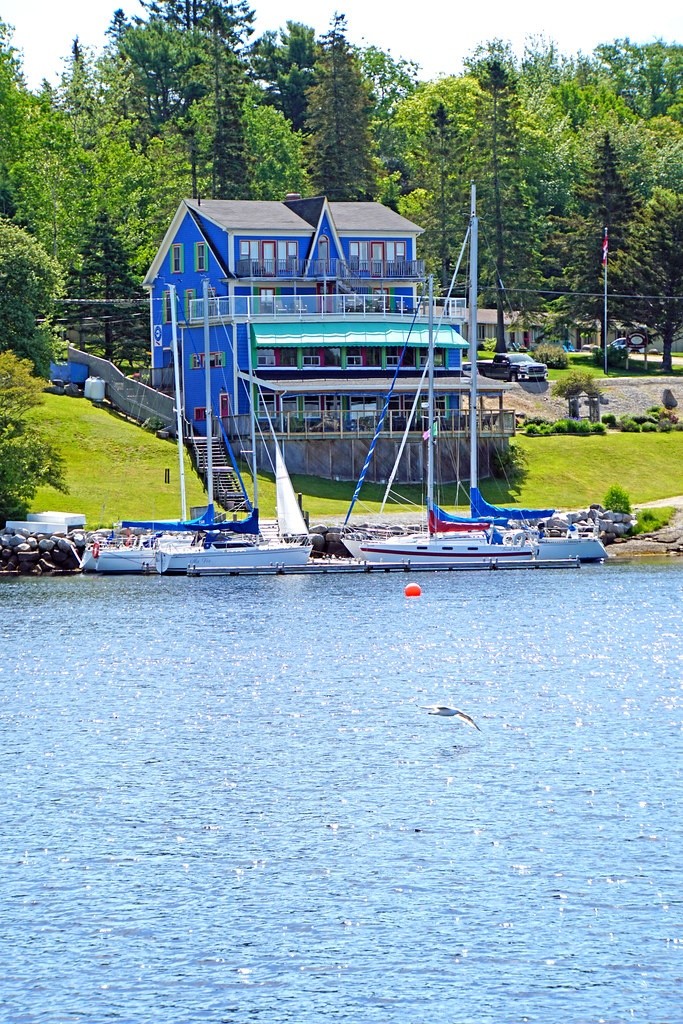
[92,542,99,558]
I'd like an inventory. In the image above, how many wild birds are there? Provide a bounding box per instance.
[415,704,482,733]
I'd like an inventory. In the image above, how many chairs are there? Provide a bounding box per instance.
[263,411,502,435]
[273,298,410,313]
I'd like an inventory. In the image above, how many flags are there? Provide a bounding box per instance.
[422,428,430,440]
[601,235,609,267]
[432,421,438,436]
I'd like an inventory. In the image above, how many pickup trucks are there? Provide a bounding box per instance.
[476,353,549,382]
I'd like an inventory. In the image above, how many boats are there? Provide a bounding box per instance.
[339,534,609,565]
[155,542,313,571]
[80,538,216,572]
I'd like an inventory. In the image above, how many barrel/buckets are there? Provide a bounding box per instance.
[85,376,96,398]
[90,377,105,400]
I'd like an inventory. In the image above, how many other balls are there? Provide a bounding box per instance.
[404,583,421,596]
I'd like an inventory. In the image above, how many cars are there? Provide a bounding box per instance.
[581,337,645,353]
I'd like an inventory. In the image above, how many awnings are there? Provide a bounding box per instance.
[251,323,471,350]
